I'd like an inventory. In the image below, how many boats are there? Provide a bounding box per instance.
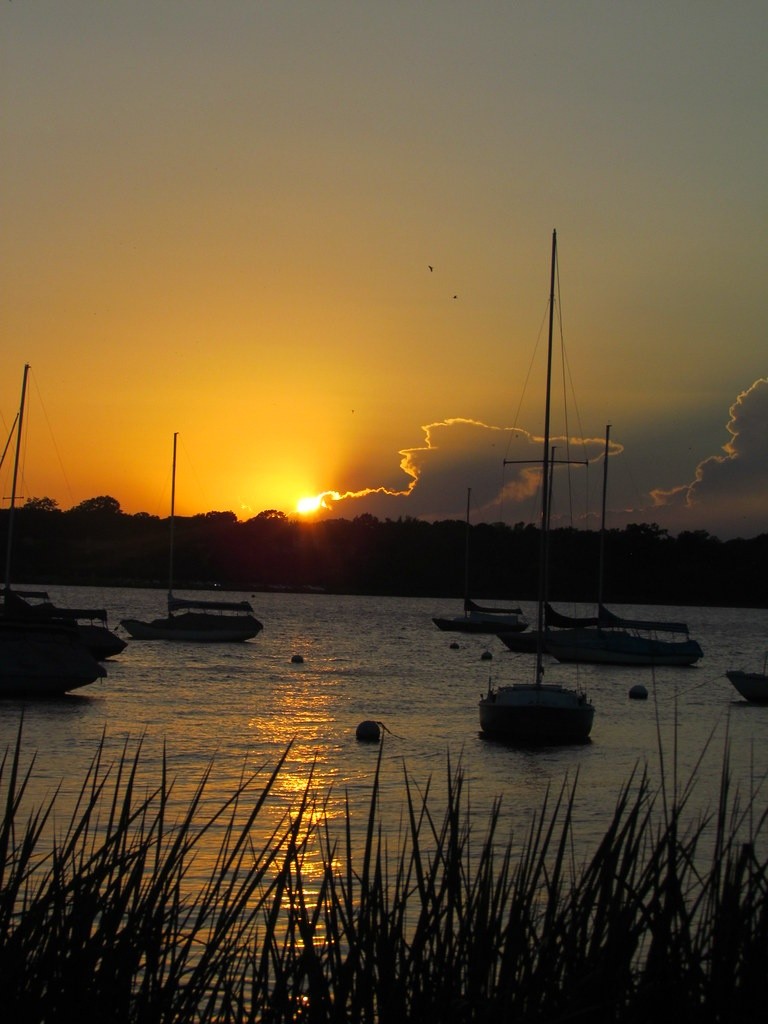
[725,671,768,705]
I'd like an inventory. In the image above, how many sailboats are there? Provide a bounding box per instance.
[494,447,629,654]
[1,364,129,703]
[477,229,599,752]
[120,431,264,643]
[431,487,530,632]
[541,424,707,668]
